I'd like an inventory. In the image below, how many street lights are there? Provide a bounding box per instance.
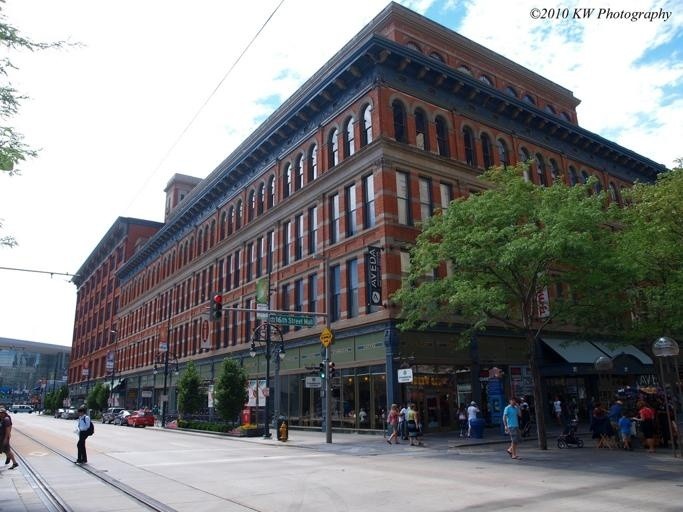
[651,336,682,458]
[152,351,178,428]
[104,368,121,409]
[47,375,90,413]
[593,356,613,409]
[247,323,283,439]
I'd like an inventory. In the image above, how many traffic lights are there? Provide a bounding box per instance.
[207,291,222,322]
[318,361,326,379]
[327,361,336,379]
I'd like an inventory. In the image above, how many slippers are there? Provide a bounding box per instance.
[507,450,521,459]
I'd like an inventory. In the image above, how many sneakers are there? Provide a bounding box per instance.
[5,458,18,469]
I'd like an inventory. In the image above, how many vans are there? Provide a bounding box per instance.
[10,405,34,414]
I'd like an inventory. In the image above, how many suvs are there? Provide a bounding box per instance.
[101,407,125,424]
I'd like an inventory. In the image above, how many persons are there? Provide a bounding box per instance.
[74,407,91,463]
[151,404,158,425]
[0,408,18,469]
[347,385,683,462]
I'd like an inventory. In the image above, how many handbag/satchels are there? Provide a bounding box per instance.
[387,413,390,425]
[417,432,423,436]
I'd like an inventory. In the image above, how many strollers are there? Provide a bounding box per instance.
[557,421,584,450]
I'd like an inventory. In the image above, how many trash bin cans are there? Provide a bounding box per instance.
[277,415,288,442]
[470,419,486,439]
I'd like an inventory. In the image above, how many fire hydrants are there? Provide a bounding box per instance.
[278,421,288,442]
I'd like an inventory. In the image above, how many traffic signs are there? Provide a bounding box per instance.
[268,315,316,326]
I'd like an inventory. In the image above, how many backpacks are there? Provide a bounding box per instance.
[84,416,94,436]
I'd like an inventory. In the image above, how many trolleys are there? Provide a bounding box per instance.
[519,422,531,437]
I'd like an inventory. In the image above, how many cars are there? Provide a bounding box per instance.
[126,410,154,428]
[60,405,79,420]
[54,408,66,418]
[113,409,135,426]
